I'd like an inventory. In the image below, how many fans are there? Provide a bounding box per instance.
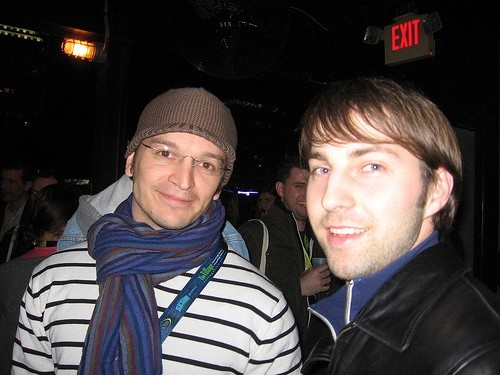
[165,0,289,80]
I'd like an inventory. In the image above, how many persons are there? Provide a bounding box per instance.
[1,183,79,375]
[252,182,276,219]
[10,87,303,375]
[238,152,346,333]
[300,79,500,375]
[0,137,500,264]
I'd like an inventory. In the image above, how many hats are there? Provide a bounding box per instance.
[126,85,239,187]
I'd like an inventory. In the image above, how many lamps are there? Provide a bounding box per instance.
[60,37,106,63]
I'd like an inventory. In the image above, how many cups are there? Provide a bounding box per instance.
[312,258,327,269]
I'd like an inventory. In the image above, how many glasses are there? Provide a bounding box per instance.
[141,141,232,176]
